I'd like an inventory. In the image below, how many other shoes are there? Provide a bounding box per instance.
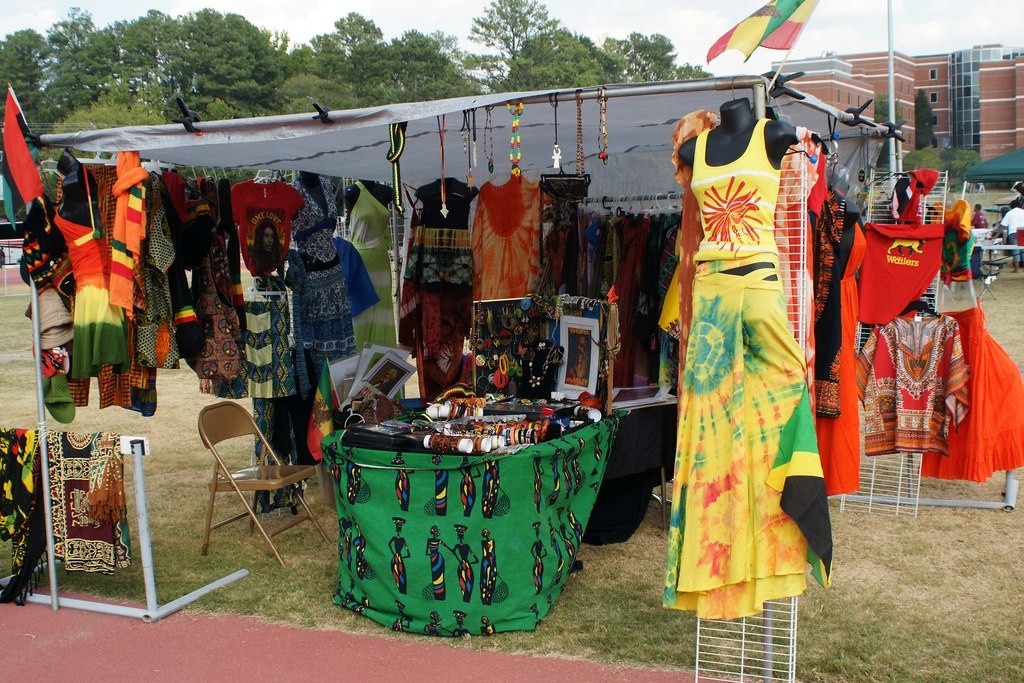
[1010,270,1018,273]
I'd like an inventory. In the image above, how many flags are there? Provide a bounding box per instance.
[707,0,818,65]
[3,91,44,202]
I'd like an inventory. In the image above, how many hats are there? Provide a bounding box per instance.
[39,288,74,423]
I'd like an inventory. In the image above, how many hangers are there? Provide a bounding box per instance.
[764,105,829,155]
[897,295,941,319]
[580,191,687,219]
[401,182,423,219]
[139,159,275,185]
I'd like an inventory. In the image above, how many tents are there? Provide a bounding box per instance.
[0,74,910,621]
[962,147,1024,200]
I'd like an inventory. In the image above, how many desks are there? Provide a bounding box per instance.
[976,244,1024,277]
[992,198,1012,223]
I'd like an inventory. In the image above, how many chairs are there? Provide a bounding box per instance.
[970,246,999,303]
[197,400,333,570]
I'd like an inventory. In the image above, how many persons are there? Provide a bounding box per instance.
[661,97,867,620]
[971,204,989,229]
[55,149,129,380]
[286,168,396,365]
[567,335,590,382]
[992,179,1024,273]
[372,369,397,395]
